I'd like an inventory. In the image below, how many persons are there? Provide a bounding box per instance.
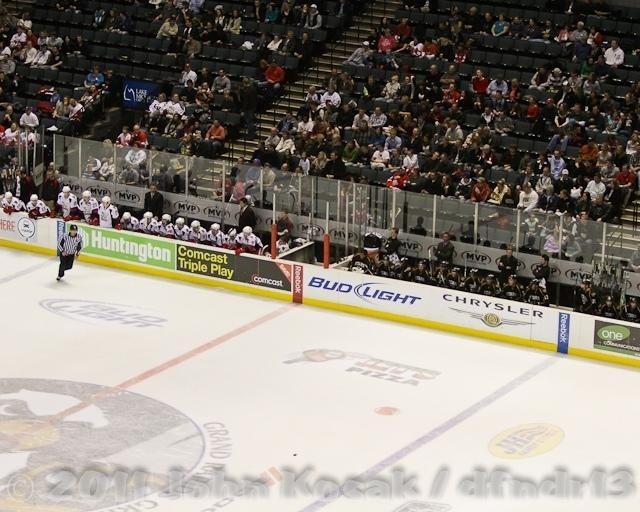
[0,0,639,323]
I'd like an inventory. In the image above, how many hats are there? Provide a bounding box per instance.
[70,225,78,231]
[562,169,568,175]
[478,177,485,182]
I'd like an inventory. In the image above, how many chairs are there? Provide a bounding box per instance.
[1,1,341,151]
[341,2,640,186]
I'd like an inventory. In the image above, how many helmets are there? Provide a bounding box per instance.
[30,194,39,202]
[418,260,539,292]
[5,191,12,198]
[83,190,91,196]
[102,196,109,203]
[62,185,71,193]
[400,257,408,263]
[143,212,252,238]
[122,211,131,219]
